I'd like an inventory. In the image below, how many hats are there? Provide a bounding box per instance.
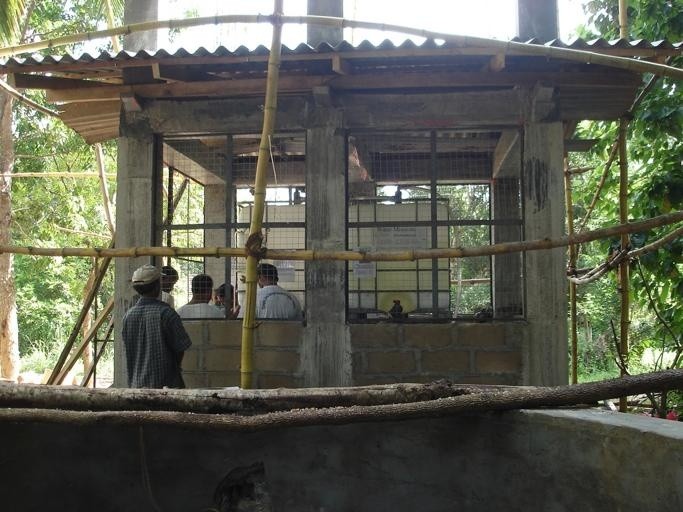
[132,262,168,286]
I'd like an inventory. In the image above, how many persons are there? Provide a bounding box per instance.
[161,263,303,319]
[120,263,192,388]
